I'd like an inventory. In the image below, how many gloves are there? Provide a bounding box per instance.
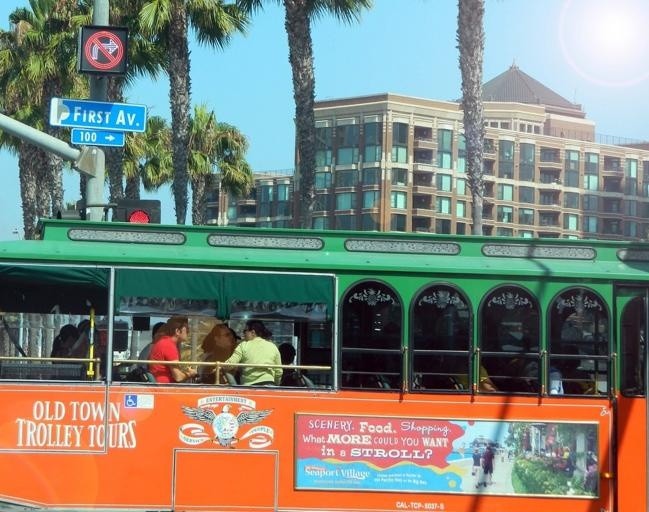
[462,432,506,456]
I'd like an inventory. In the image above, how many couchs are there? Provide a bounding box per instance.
[113,198,162,226]
[94,317,129,356]
[49,199,85,220]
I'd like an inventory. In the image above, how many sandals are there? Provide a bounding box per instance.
[0,221,649,511]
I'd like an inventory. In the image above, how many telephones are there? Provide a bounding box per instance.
[48,96,149,134]
[70,127,125,148]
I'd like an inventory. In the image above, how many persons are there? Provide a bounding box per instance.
[50,315,306,387]
[469,434,598,492]
[456,340,602,397]
